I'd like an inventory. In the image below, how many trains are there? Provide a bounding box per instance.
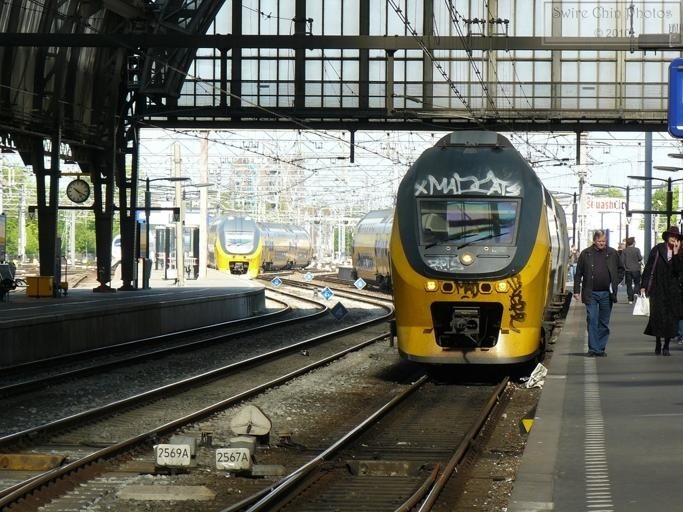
[214,213,312,279]
[351,130,570,364]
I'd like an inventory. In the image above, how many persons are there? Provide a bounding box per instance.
[569,245,580,281]
[618,226,683,356]
[573,231,625,357]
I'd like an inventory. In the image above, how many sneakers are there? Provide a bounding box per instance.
[586,350,607,358]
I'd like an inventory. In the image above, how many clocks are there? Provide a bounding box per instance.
[65,179,91,203]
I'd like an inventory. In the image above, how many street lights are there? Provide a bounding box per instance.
[627,176,682,229]
[138,177,191,258]
[590,184,664,247]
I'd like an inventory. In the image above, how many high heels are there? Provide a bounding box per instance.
[655,342,671,356]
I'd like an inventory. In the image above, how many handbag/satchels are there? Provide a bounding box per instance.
[632,293,651,317]
